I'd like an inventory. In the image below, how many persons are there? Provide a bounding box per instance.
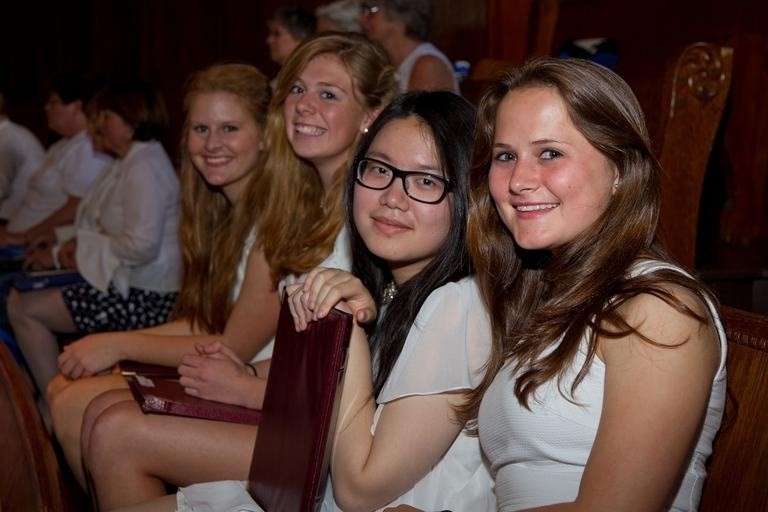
[383,57,729,511]
[259,0,457,99]
[46,31,496,509]
[0,60,183,399]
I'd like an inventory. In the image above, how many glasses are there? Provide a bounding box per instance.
[354,158,454,204]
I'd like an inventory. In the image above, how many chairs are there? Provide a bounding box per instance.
[0,338,64,512]
